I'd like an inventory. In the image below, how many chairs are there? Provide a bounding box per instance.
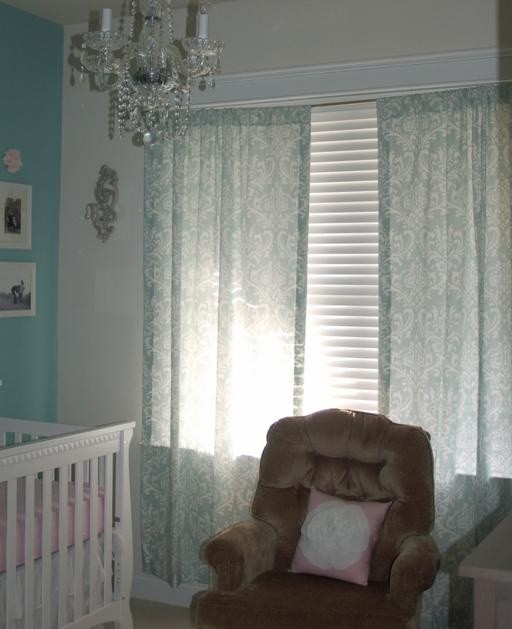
[189,408,442,628]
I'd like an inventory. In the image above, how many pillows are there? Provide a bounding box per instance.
[287,485,393,587]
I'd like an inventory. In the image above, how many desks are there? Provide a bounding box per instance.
[457,515,512,628]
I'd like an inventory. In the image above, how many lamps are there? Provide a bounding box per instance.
[79,1,225,152]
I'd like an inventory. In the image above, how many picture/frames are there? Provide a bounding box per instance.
[0,181,33,250]
[1,261,37,317]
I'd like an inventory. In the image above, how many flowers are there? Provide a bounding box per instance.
[3,148,22,173]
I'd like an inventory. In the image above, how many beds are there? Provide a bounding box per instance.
[0,416,137,629]
